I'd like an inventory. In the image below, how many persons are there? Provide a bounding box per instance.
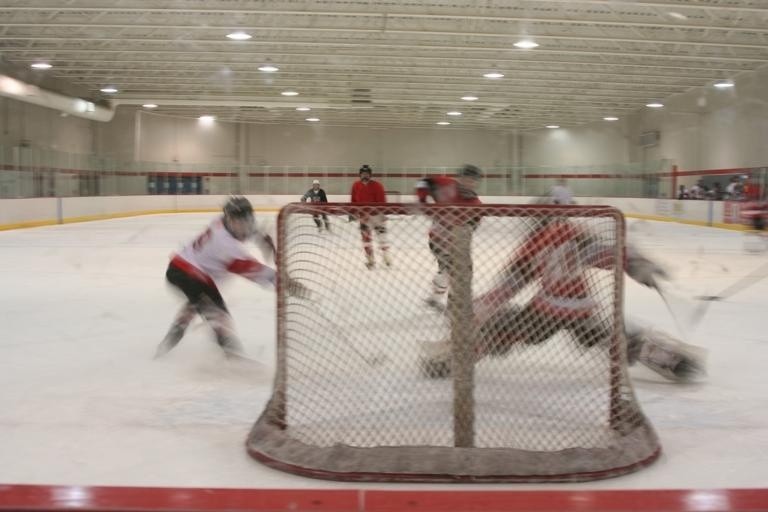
[552,178,578,204]
[300,179,329,230]
[349,165,392,270]
[415,164,483,311]
[159,197,304,361]
[677,174,744,201]
[424,185,697,381]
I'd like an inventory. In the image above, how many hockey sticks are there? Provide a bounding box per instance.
[695,267,768,300]
[311,305,392,367]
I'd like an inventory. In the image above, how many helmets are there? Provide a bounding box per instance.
[313,179,320,183]
[224,197,252,218]
[359,165,371,176]
